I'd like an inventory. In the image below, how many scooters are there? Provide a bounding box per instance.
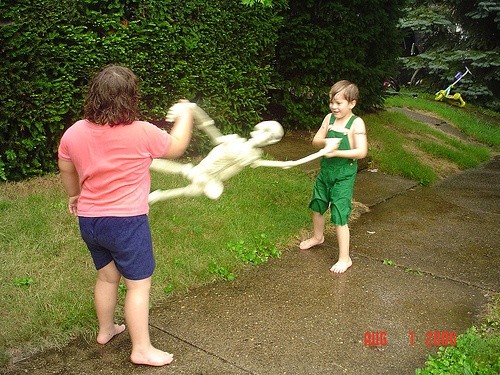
[435,65,474,108]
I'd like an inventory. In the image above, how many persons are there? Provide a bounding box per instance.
[298,81,367,274]
[58,66,196,367]
[146,98,343,206]
